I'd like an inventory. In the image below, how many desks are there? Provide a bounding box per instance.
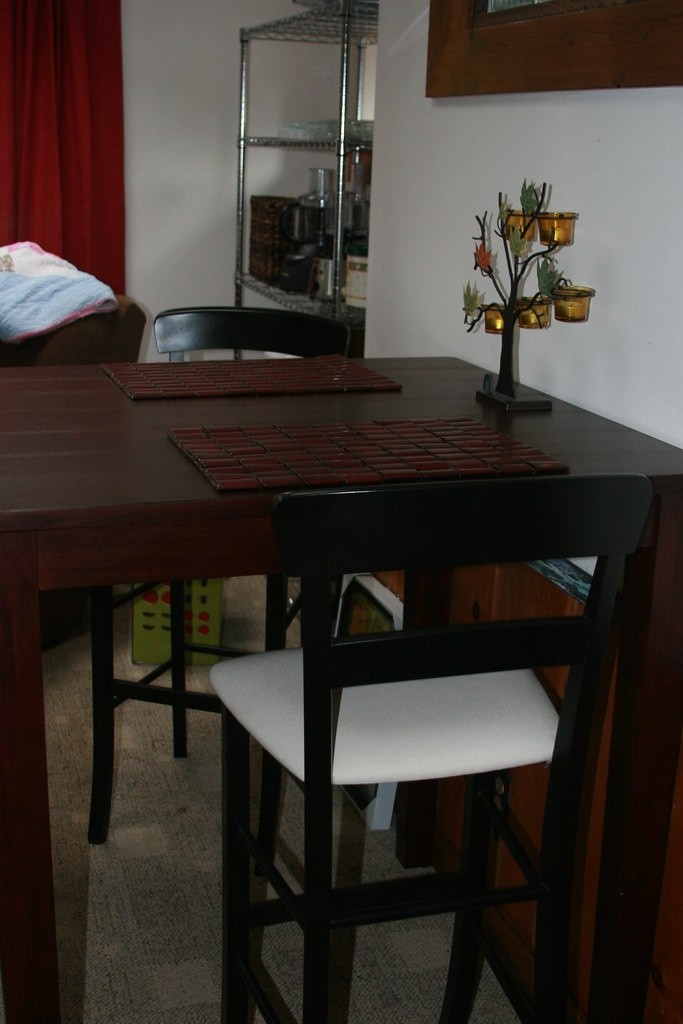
[1,356,683,1024]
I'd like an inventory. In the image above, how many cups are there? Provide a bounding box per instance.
[303,118,373,143]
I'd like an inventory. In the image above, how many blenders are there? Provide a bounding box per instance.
[299,168,369,309]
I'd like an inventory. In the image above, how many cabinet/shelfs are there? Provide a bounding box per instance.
[233,0,379,360]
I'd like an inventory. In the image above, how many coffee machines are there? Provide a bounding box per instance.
[278,201,326,292]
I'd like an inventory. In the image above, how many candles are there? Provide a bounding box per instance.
[552,286,595,322]
[505,211,534,240]
[515,295,551,330]
[537,212,578,245]
[483,303,506,331]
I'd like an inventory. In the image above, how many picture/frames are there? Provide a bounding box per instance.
[333,572,403,834]
[425,0,683,98]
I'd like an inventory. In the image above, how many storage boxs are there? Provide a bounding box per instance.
[128,580,224,666]
[248,194,294,286]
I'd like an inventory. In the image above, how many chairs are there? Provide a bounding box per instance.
[87,307,349,846]
[211,472,655,1024]
